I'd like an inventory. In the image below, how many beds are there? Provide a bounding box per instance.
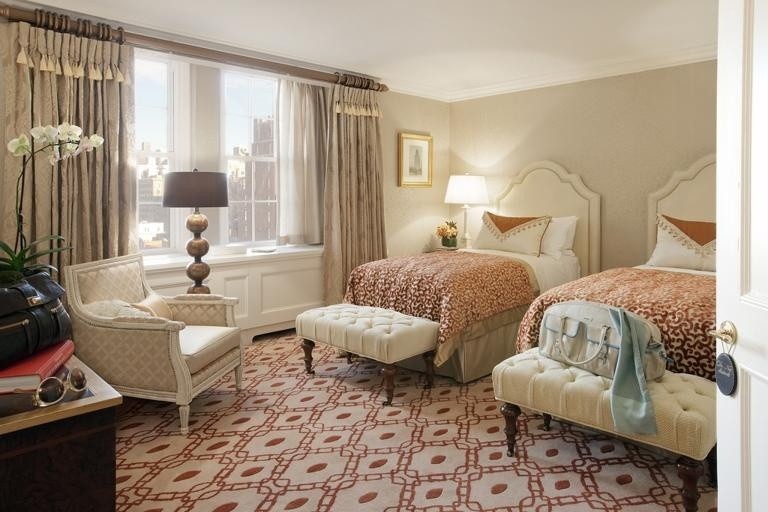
[513,153,717,436]
[340,160,602,388]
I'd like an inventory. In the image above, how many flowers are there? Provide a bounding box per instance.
[437,220,459,239]
[0,114,108,282]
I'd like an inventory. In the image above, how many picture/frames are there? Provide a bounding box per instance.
[397,130,434,189]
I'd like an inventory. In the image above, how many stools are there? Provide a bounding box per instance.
[293,301,445,404]
[489,347,717,511]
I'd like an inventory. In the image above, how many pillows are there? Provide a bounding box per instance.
[643,211,716,271]
[541,214,575,259]
[561,215,579,257]
[470,209,552,260]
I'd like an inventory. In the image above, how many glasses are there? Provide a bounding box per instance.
[32,368,89,409]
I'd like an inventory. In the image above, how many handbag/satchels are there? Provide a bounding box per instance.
[0,271,73,370]
[537,300,669,383]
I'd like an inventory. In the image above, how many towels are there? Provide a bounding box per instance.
[605,306,658,437]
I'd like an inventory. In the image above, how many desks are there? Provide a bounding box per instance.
[0,354,127,511]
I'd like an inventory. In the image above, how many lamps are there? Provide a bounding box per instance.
[161,165,230,294]
[444,173,490,248]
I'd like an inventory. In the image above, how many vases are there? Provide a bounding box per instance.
[441,237,458,247]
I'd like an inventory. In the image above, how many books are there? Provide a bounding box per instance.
[0,339,75,394]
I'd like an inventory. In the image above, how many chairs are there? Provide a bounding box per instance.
[64,252,248,433]
